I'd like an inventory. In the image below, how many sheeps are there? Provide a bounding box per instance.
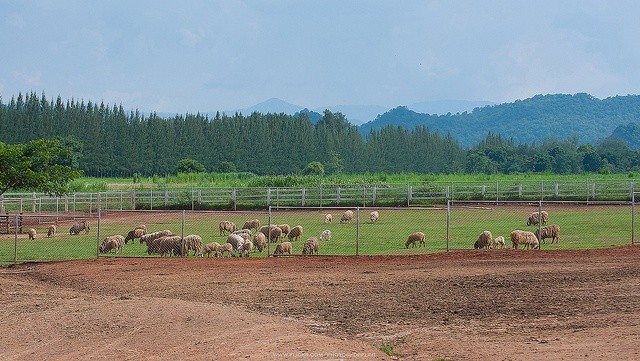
[99,236,125,255]
[509,230,539,251]
[173,234,203,257]
[47,225,56,238]
[147,234,180,256]
[325,214,332,225]
[253,233,267,254]
[215,243,234,259]
[534,225,561,245]
[125,230,145,245]
[219,220,238,237]
[239,234,253,258]
[341,210,354,224]
[278,224,290,241]
[493,236,506,249]
[158,237,184,257]
[201,242,221,259]
[473,231,492,251]
[232,229,252,238]
[527,211,549,227]
[69,220,91,236]
[140,230,173,247]
[134,225,148,235]
[404,232,426,250]
[302,237,319,257]
[369,212,379,224]
[268,228,284,244]
[226,234,244,258]
[259,225,276,238]
[319,230,332,242]
[287,225,303,242]
[273,242,292,258]
[242,220,260,232]
[28,228,36,241]
[103,240,118,255]
